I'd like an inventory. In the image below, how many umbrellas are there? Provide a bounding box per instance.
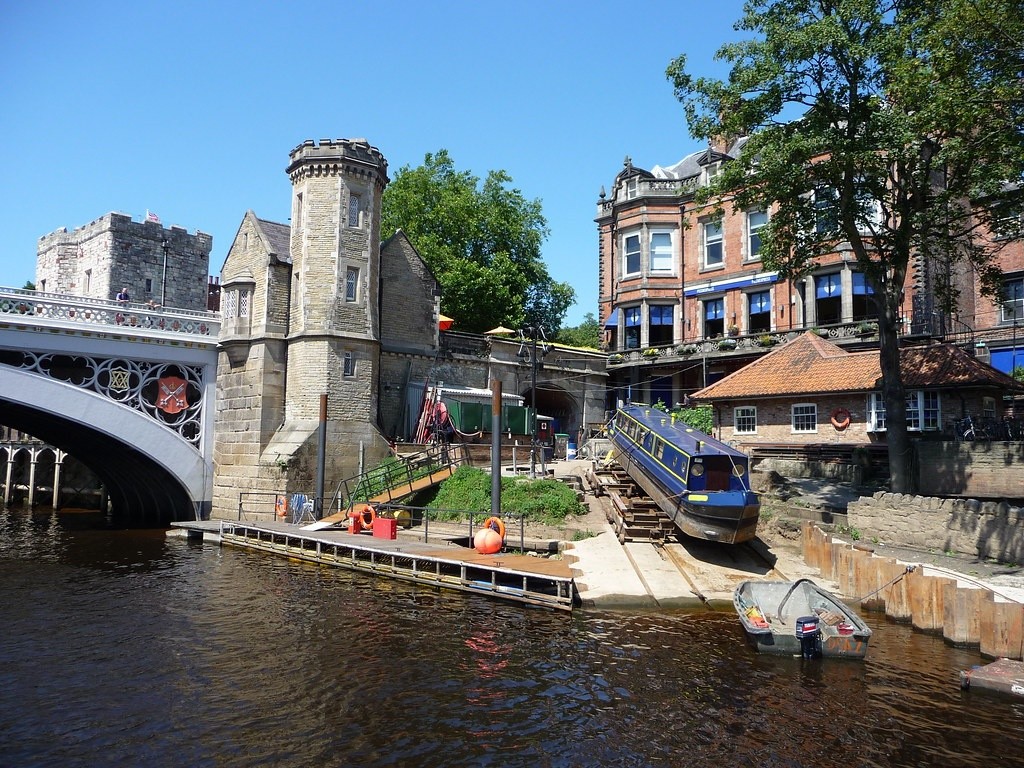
[484,325,515,338]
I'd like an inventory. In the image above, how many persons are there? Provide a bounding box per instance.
[146,300,161,308]
[117,288,130,302]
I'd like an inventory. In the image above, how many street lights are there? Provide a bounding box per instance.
[159,236,170,305]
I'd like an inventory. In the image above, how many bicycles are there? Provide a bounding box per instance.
[940,405,1024,443]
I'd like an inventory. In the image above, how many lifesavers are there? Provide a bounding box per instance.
[277,495,288,516]
[830,407,852,430]
[484,516,505,540]
[359,505,377,530]
[432,402,448,426]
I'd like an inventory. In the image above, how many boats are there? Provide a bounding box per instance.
[734,574,874,661]
[607,399,763,550]
